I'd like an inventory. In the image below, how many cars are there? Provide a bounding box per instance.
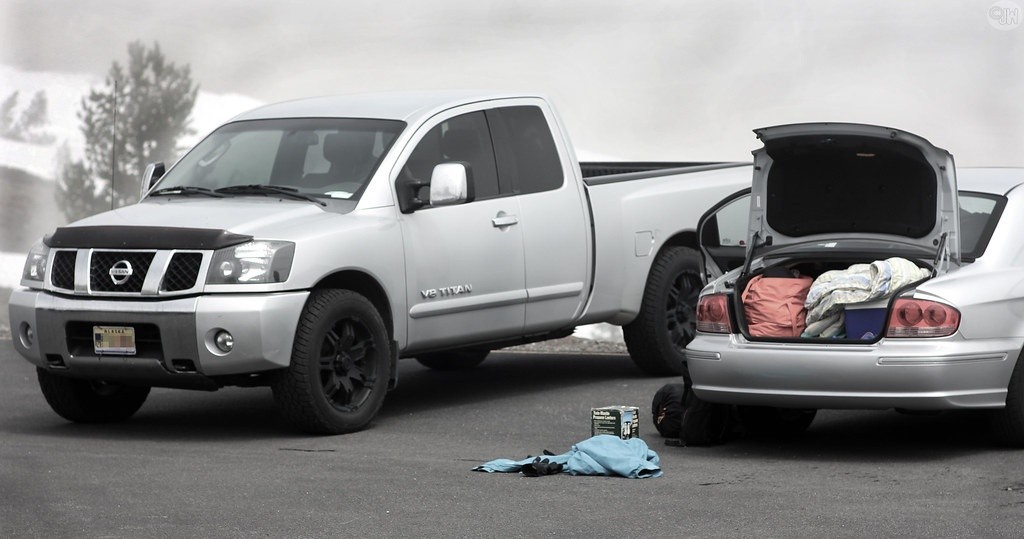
[681,123,1024,451]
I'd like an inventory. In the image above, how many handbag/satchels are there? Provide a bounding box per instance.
[741,273,812,339]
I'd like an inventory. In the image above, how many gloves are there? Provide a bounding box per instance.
[526,449,556,459]
[521,456,563,477]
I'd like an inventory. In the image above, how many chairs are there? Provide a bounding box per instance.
[300,134,366,188]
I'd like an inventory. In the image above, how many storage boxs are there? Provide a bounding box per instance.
[843,297,890,340]
[591,405,640,440]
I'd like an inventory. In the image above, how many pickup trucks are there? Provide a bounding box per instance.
[9,94,769,439]
